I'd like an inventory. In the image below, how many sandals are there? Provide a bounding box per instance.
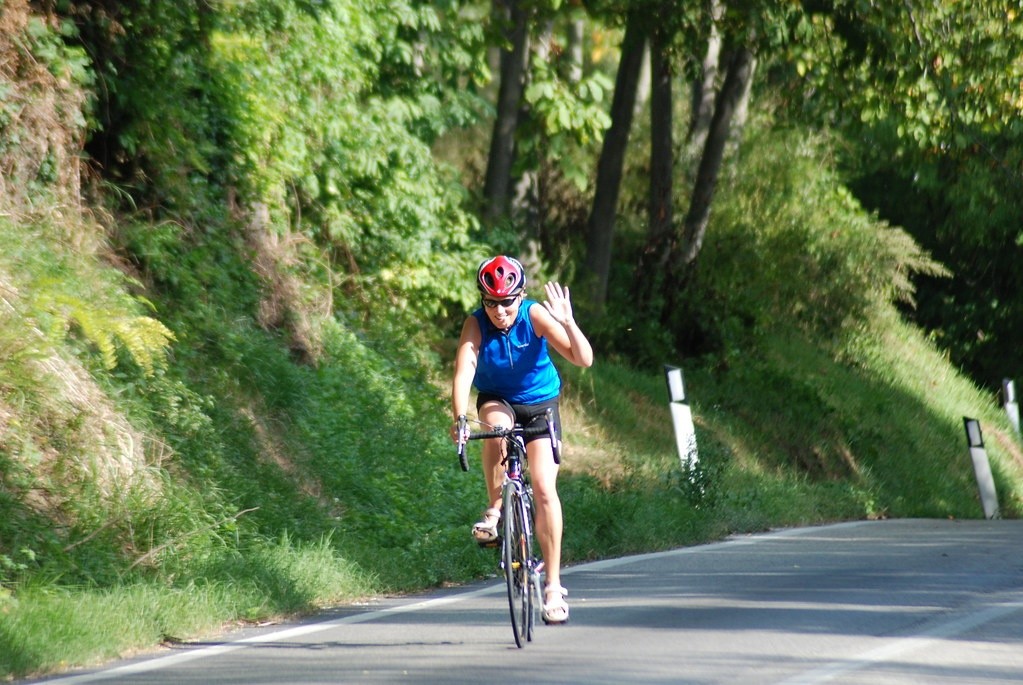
[470,508,501,542]
[543,585,569,622]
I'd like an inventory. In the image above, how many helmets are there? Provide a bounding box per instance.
[477,255,526,296]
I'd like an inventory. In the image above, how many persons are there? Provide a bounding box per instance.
[449,255,594,625]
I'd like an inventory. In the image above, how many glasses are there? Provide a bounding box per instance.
[482,295,519,308]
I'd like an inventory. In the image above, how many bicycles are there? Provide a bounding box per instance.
[455,415,568,649]
